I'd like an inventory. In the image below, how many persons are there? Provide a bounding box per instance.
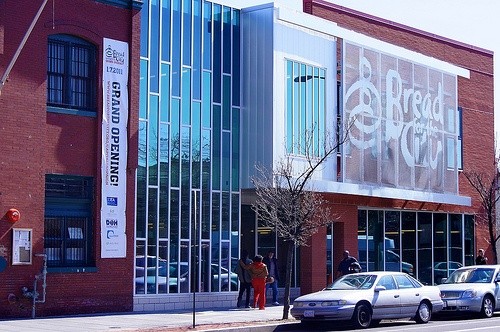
[237,251,253,308]
[475,249,488,265]
[338,250,360,276]
[264,250,280,305]
[240,255,268,310]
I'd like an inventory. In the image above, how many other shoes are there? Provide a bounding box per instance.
[273,302,279,305]
[246,306,252,309]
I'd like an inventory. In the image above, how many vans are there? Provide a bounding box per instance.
[325,235,414,275]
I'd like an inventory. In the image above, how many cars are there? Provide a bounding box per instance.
[135,254,169,276]
[436,265,500,318]
[425,261,463,280]
[289,270,445,330]
[136,261,239,294]
